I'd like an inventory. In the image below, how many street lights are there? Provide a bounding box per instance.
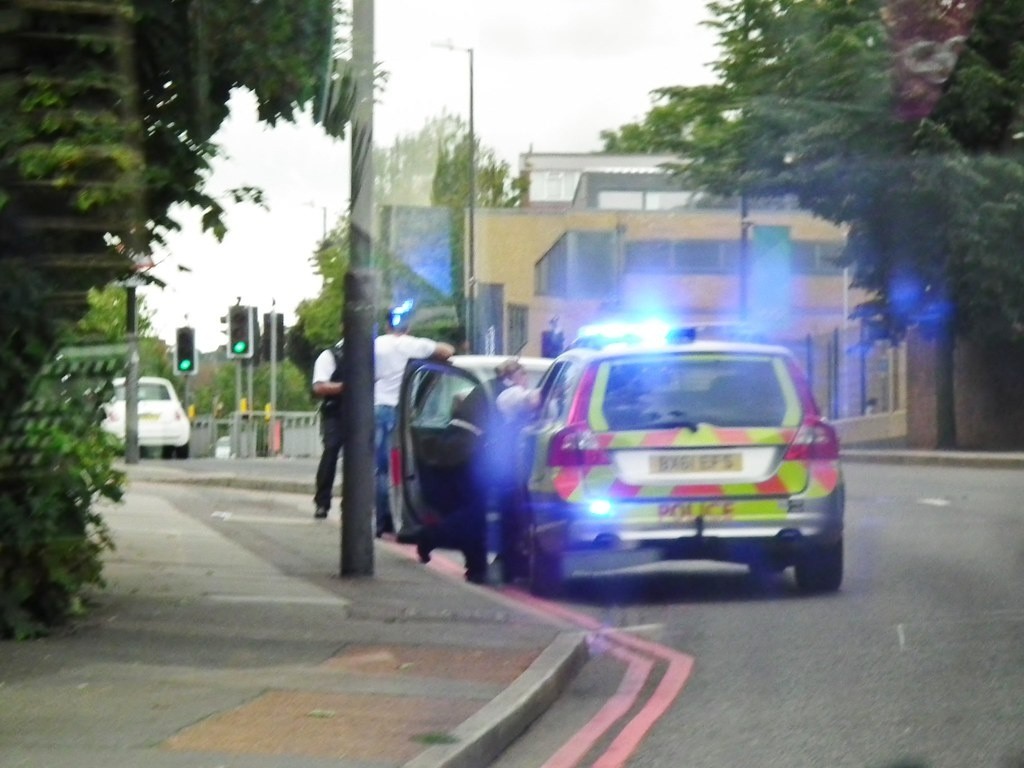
[440,40,474,354]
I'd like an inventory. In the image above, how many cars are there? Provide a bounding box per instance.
[209,436,236,459]
[96,376,191,460]
[445,355,556,395]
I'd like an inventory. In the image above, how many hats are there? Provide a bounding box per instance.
[494,360,521,380]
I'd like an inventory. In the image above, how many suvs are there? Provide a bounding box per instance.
[387,324,846,598]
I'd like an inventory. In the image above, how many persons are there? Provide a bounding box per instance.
[416,359,543,590]
[372,305,454,544]
[310,307,355,519]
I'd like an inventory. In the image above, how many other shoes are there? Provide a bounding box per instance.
[315,505,327,517]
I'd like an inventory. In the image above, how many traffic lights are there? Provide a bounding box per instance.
[229,306,249,353]
[219,315,229,354]
[176,326,193,371]
